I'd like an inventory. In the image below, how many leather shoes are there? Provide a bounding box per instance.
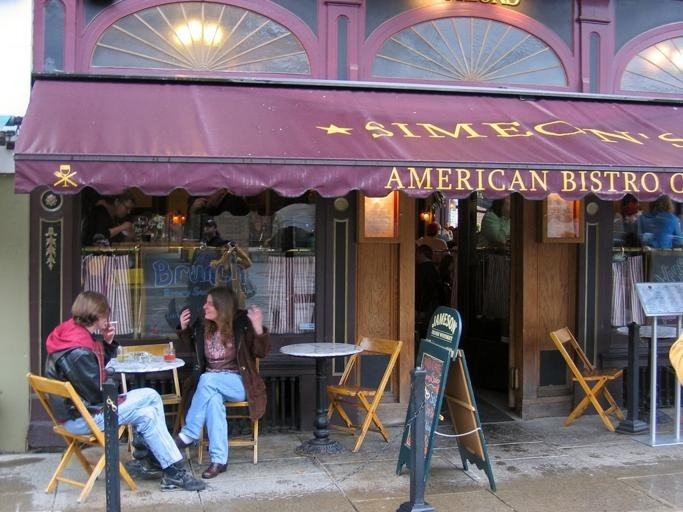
[202,462,227,478]
[174,436,194,449]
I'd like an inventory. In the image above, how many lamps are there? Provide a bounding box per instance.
[418,206,431,221]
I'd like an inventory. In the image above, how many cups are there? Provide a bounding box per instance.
[164,340,176,362]
[117,347,129,363]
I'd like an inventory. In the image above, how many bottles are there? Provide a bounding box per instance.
[170,217,182,245]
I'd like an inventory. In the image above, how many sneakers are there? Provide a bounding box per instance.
[141,466,205,490]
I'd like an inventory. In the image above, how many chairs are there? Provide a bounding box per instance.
[197,356,261,465]
[119,341,191,461]
[550,326,627,433]
[27,372,139,505]
[327,335,404,455]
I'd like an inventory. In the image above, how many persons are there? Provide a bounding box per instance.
[187,184,316,250]
[83,190,135,246]
[172,287,270,479]
[45,290,206,491]
[476,196,511,250]
[634,195,683,250]
[613,193,646,233]
[412,220,458,316]
